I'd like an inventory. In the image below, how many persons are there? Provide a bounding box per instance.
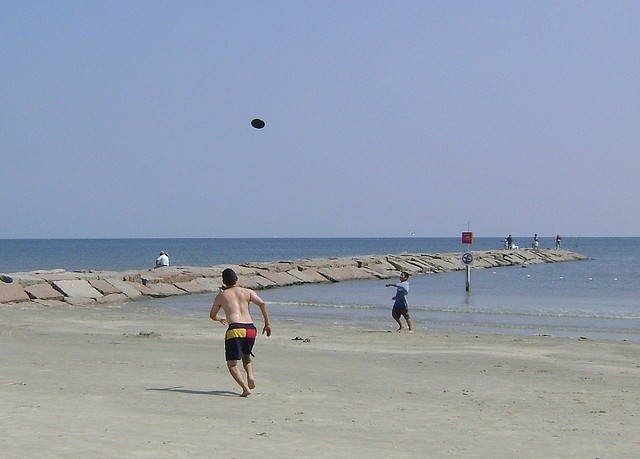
[506,234,514,249]
[553,233,562,248]
[504,237,509,249]
[209,267,273,397]
[533,232,539,252]
[384,271,414,335]
[153,248,170,267]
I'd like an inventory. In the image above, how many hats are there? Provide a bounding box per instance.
[159,251,164,254]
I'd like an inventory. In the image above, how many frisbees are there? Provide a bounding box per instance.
[249,118,266,130]
